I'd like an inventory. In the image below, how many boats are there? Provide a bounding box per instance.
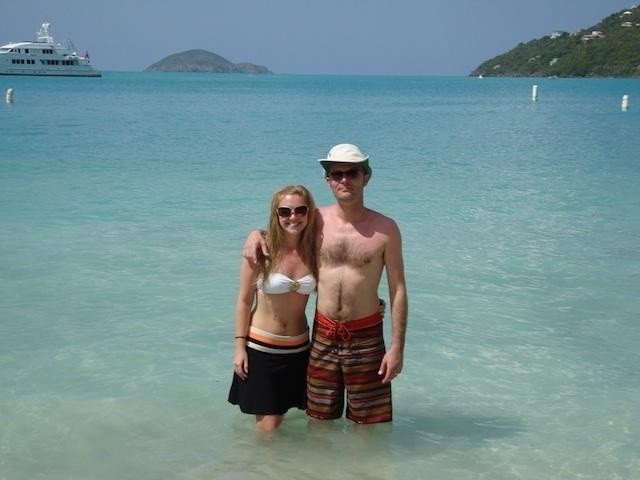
[0,19,102,77]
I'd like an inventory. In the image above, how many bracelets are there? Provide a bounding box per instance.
[235,336,247,339]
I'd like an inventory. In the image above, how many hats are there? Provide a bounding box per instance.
[317,144,370,175]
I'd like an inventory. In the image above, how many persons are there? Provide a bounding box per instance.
[243,143,408,430]
[227,183,386,441]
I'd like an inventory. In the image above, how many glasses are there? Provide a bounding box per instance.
[274,206,310,217]
[326,168,366,182]
